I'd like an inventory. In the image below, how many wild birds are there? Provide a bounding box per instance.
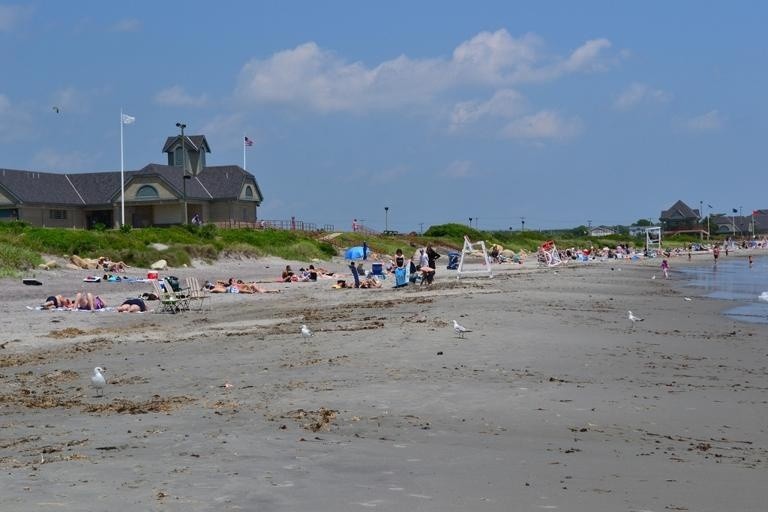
[299,324,314,345]
[651,274,656,280]
[684,296,692,301]
[627,310,644,326]
[90,366,109,397]
[452,320,473,339]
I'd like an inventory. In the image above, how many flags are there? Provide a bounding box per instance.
[752,210,761,217]
[121,113,135,126]
[244,136,253,146]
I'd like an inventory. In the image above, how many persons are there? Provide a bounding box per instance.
[661,259,670,278]
[447,254,459,270]
[349,240,441,289]
[351,218,358,232]
[291,217,296,230]
[331,279,384,289]
[488,235,767,265]
[194,213,200,225]
[282,265,342,282]
[260,220,264,229]
[42,253,280,315]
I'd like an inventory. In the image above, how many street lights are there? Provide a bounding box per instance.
[469,218,472,229]
[384,207,388,233]
[176,123,189,225]
[699,201,703,218]
[521,221,524,231]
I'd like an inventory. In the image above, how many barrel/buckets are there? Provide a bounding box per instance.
[147,270,159,280]
[371,263,384,275]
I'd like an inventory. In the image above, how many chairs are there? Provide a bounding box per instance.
[147,274,213,315]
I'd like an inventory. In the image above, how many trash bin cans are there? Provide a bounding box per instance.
[446,252,461,269]
